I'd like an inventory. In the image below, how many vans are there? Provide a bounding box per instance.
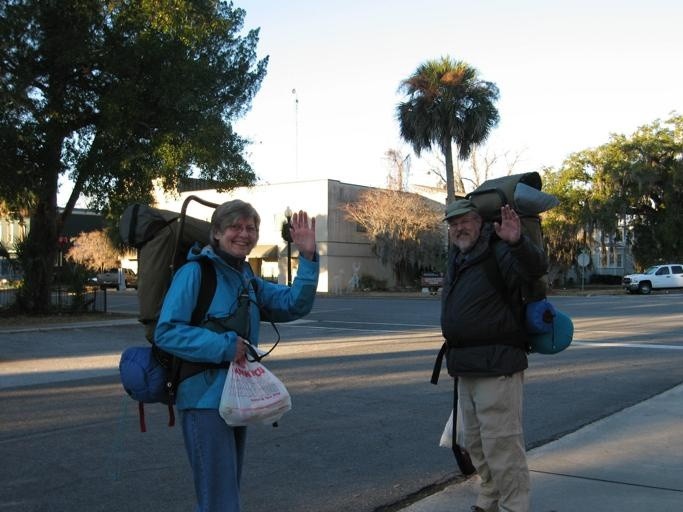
[621,263,683,295]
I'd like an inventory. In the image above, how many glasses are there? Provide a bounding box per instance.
[226,224,259,234]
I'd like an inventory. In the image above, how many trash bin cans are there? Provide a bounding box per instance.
[560,290,568,296]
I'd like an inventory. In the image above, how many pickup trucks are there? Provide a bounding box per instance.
[418,270,445,292]
[97,267,138,291]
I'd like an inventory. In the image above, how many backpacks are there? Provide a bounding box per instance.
[118,197,261,347]
[459,169,551,310]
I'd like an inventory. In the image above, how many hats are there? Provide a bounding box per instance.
[440,199,477,222]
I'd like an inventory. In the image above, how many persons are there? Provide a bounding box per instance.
[152,197,319,512]
[438,196,550,512]
